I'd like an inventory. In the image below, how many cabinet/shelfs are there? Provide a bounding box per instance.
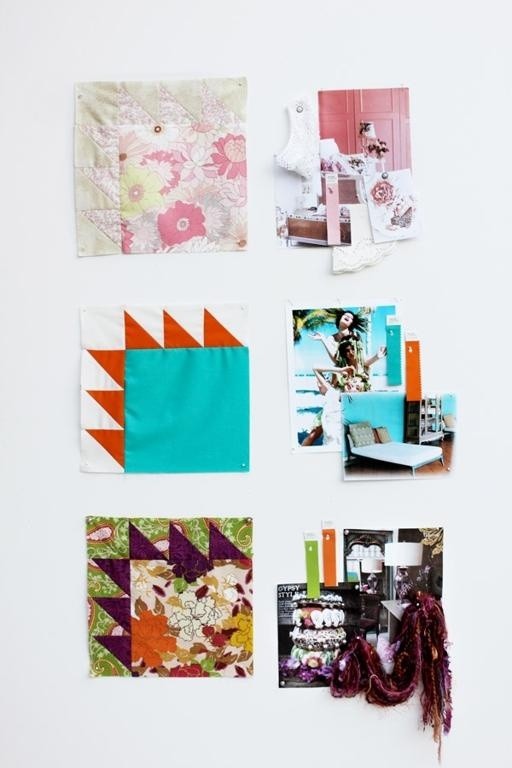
[317,87,410,202]
[405,393,445,447]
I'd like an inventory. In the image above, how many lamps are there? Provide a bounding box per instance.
[361,558,382,594]
[383,539,423,599]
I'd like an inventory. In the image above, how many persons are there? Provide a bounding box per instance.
[325,308,368,358]
[301,366,367,446]
[307,329,388,383]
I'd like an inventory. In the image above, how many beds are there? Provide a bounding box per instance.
[346,427,445,474]
